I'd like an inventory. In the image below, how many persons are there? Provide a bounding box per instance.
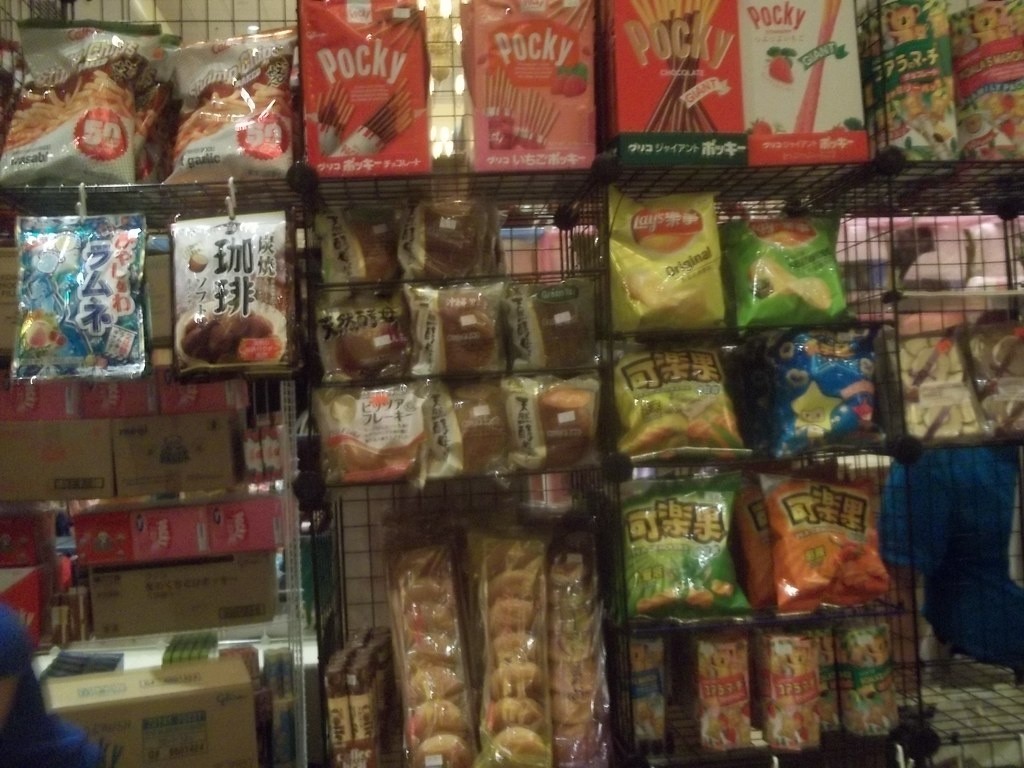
[877,307,1024,695]
[0,606,104,768]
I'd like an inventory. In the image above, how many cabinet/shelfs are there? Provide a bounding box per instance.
[0,0,1024,768]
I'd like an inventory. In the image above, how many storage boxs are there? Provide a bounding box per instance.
[617,616,900,758]
[460,0,594,171]
[738,0,869,165]
[298,1,433,178]
[42,654,258,768]
[851,0,1023,163]
[2,245,284,640]
[594,1,746,168]
